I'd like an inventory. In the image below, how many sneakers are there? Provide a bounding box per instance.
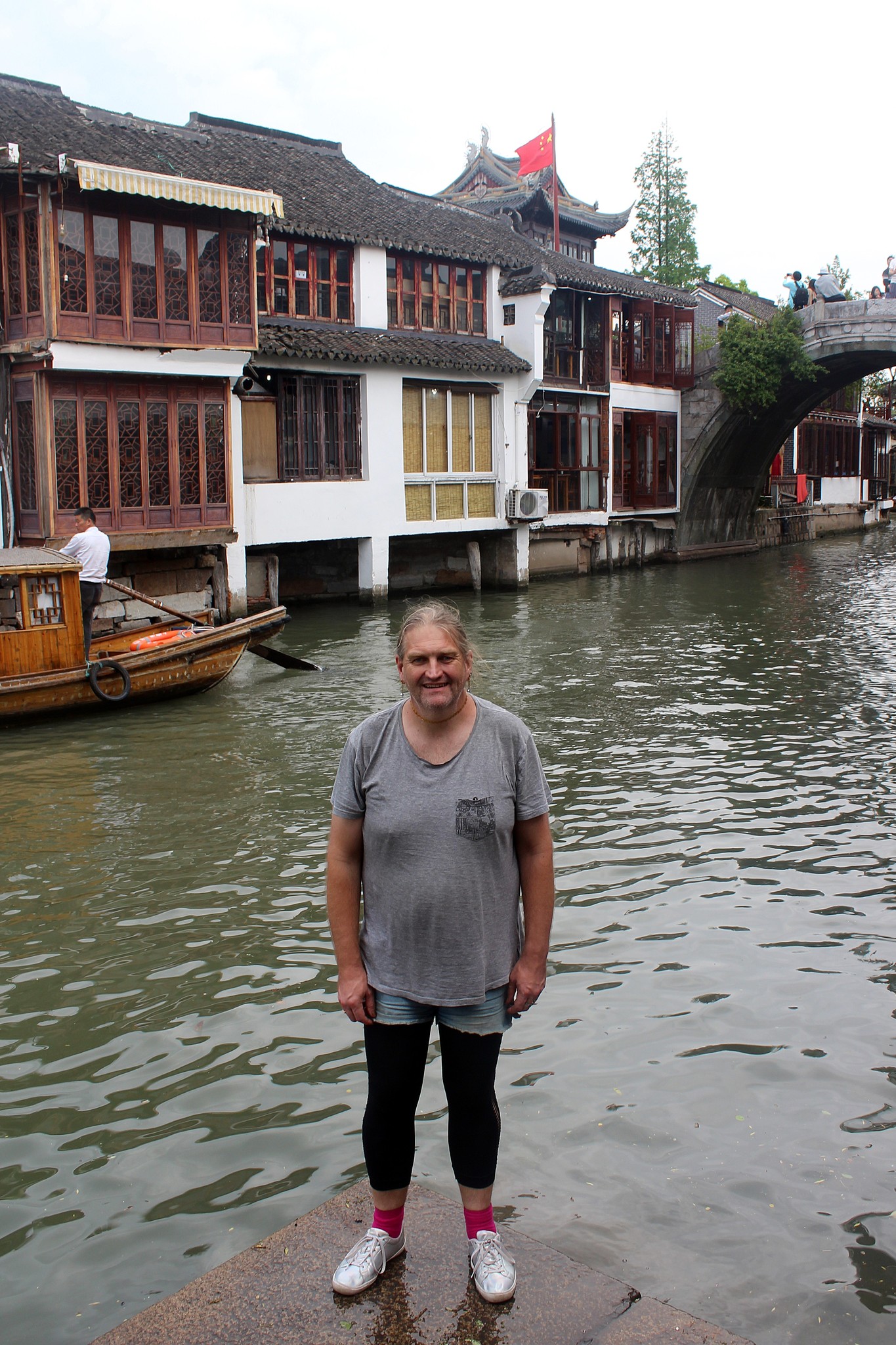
[331,1225,407,1295]
[466,1230,519,1304]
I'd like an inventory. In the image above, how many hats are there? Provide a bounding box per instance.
[817,267,830,275]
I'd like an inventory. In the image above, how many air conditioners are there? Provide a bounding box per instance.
[507,487,549,519]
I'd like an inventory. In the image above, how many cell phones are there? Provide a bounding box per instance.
[787,273,792,276]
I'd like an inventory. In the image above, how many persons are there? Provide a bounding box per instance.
[783,268,847,313]
[59,507,111,662]
[717,304,739,337]
[871,256,896,299]
[326,602,554,1303]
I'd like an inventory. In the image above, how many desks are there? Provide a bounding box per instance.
[533,473,570,511]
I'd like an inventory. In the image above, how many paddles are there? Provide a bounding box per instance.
[103,579,323,672]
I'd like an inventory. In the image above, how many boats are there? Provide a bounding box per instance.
[0,546,292,718]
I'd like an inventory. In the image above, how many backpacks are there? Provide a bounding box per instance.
[790,281,809,306]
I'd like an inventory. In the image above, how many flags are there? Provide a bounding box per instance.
[515,128,554,181]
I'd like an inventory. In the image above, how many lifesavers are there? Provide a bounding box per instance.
[130,629,195,651]
